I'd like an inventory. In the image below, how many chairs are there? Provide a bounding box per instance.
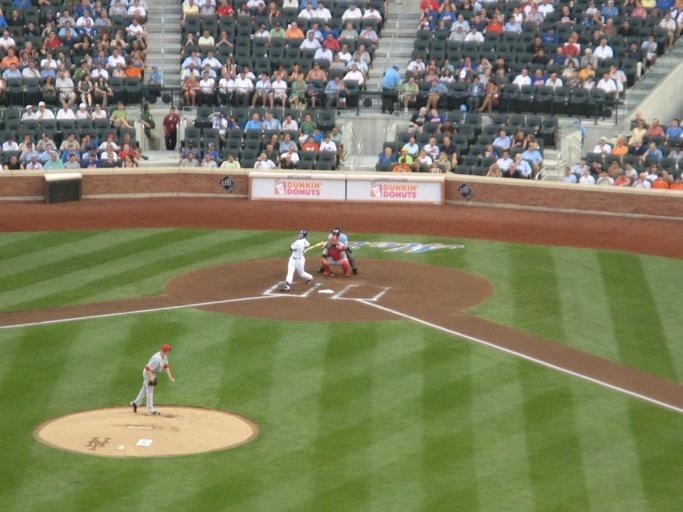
[1,1,683,187]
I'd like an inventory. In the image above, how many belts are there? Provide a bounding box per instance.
[293,256,301,259]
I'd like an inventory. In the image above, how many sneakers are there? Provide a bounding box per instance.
[280,285,291,291]
[319,267,359,278]
[305,277,313,285]
[130,401,137,413]
[147,411,160,416]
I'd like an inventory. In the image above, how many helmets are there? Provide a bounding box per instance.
[161,344,171,352]
[299,230,307,238]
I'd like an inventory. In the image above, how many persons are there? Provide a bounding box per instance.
[376,0,682,188]
[130,344,177,416]
[318,227,358,277]
[0,1,160,170]
[163,0,388,171]
[280,231,313,292]
